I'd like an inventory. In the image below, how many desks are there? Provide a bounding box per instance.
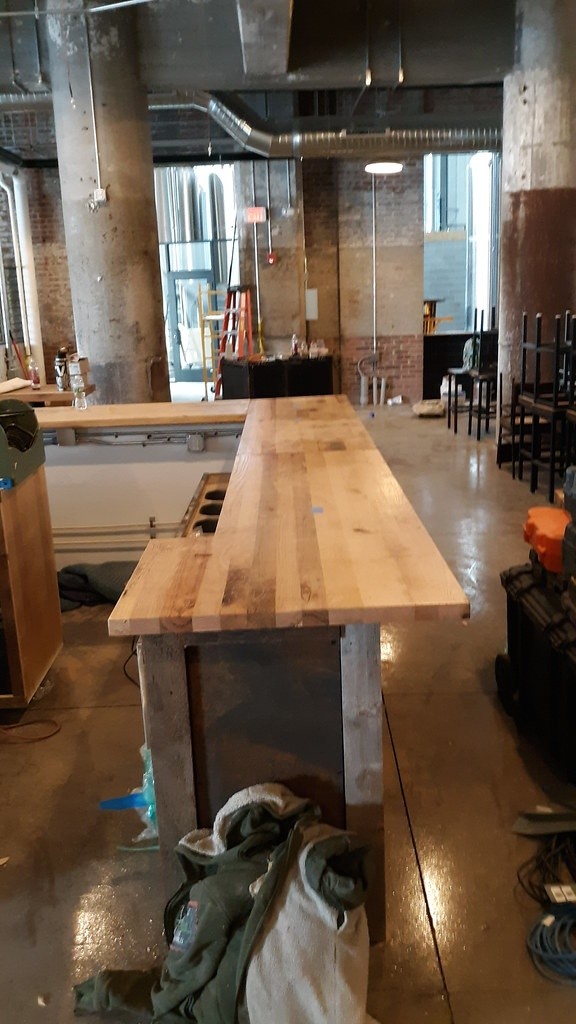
[32,394,471,944]
[0,380,95,406]
[220,350,334,399]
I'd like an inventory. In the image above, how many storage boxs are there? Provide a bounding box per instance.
[68,356,98,407]
[497,506,576,785]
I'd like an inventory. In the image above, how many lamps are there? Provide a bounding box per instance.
[364,155,404,175]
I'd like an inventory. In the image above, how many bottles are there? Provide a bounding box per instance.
[54,350,70,392]
[72,373,87,410]
[291,333,299,357]
[300,341,308,360]
[29,360,41,391]
[309,338,327,360]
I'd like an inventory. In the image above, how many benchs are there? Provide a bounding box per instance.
[448,307,576,503]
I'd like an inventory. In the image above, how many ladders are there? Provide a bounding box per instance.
[214,285,253,401]
